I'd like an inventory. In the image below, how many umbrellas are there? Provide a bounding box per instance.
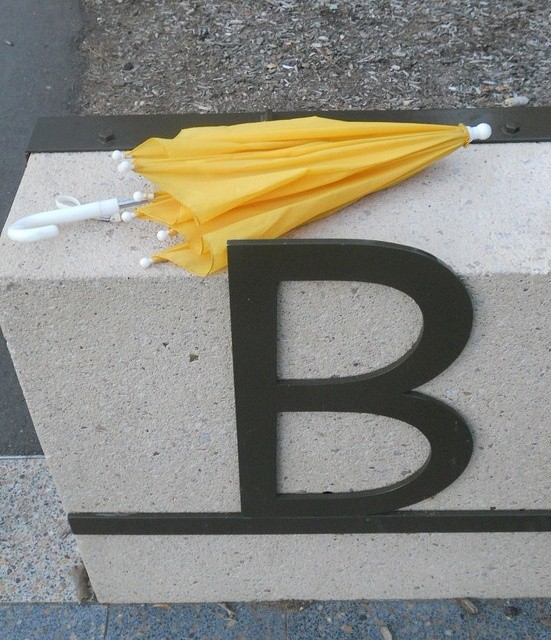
[8,115,492,277]
